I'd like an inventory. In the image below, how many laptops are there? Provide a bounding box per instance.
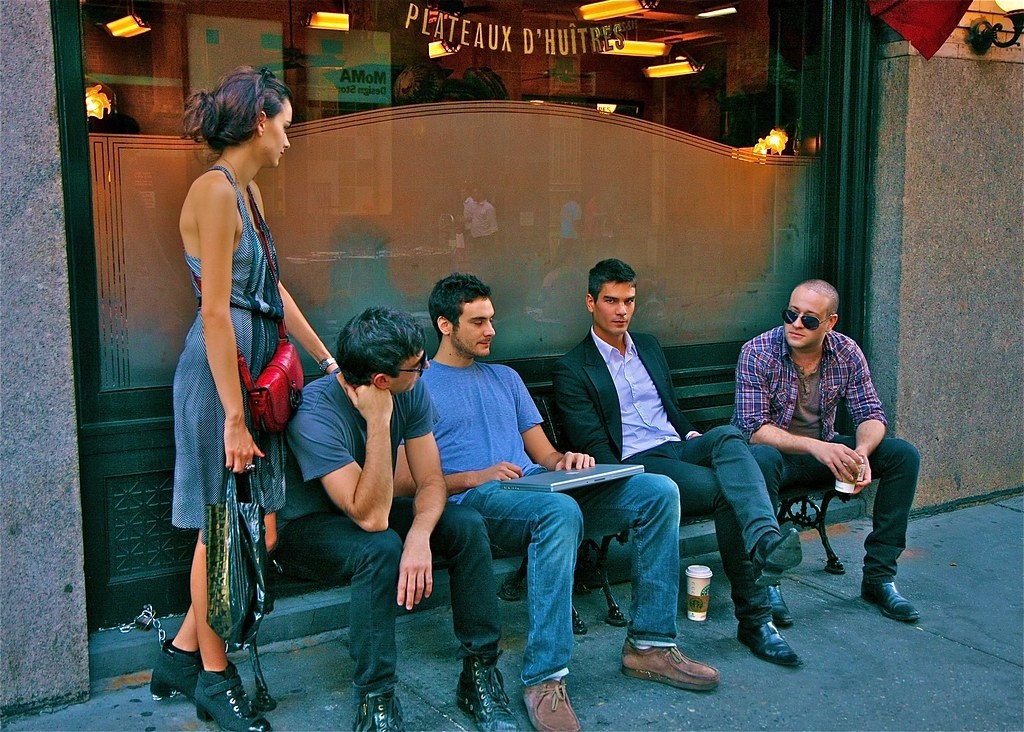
[500,463,644,492]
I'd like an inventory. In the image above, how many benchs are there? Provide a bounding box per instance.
[229,339,846,712]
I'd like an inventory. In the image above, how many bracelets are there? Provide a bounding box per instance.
[319,358,336,372]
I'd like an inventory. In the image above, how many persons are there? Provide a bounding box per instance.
[730,281,920,625]
[553,258,803,666]
[394,276,721,732]
[151,73,339,732]
[280,308,520,732]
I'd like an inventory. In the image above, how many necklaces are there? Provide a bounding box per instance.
[223,157,240,186]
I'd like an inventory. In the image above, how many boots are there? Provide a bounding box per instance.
[456,648,520,732]
[353,687,403,732]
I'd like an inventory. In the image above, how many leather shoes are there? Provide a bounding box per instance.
[769,588,793,625]
[737,616,800,668]
[751,528,802,587]
[861,579,921,621]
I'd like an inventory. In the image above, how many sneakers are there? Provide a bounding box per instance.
[524,676,581,732]
[622,639,721,692]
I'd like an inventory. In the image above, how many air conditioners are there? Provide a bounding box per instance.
[753,117,801,159]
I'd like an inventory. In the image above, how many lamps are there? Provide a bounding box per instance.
[966,0,1024,56]
[641,42,706,80]
[597,33,673,60]
[300,10,351,31]
[571,0,661,24]
[103,15,151,39]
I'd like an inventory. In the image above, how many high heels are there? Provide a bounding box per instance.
[194,662,270,732]
[150,638,203,702]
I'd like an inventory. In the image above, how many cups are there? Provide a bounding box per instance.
[835,454,857,494]
[685,564,713,622]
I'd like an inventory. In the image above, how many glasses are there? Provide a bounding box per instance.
[781,307,833,331]
[393,351,427,379]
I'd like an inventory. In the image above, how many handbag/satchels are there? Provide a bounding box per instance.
[205,466,273,648]
[247,342,305,433]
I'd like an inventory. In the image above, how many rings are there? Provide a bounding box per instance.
[244,463,255,472]
